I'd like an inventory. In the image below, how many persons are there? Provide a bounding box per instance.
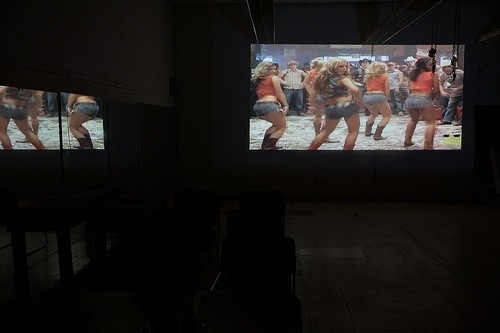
[251,56,464,143]
[306,57,364,150]
[252,58,288,150]
[363,61,392,141]
[46,91,100,149]
[405,57,444,149]
[0,86,45,151]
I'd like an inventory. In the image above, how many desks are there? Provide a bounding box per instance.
[10,200,101,288]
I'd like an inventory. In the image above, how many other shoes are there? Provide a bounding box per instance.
[307,140,322,150]
[398,111,404,115]
[436,120,452,126]
[46,112,54,117]
[454,120,462,126]
[342,141,355,150]
[297,112,306,116]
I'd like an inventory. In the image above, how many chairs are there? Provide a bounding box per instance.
[42,188,304,333]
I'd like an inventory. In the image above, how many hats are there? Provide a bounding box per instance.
[387,61,395,66]
[403,56,415,61]
[288,60,299,66]
[358,58,372,67]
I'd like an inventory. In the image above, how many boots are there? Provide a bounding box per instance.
[365,121,373,136]
[71,136,92,149]
[423,133,434,150]
[404,130,415,147]
[261,134,283,148]
[261,133,276,150]
[16,122,40,143]
[312,122,340,143]
[321,123,331,137]
[374,124,387,140]
[83,132,94,147]
